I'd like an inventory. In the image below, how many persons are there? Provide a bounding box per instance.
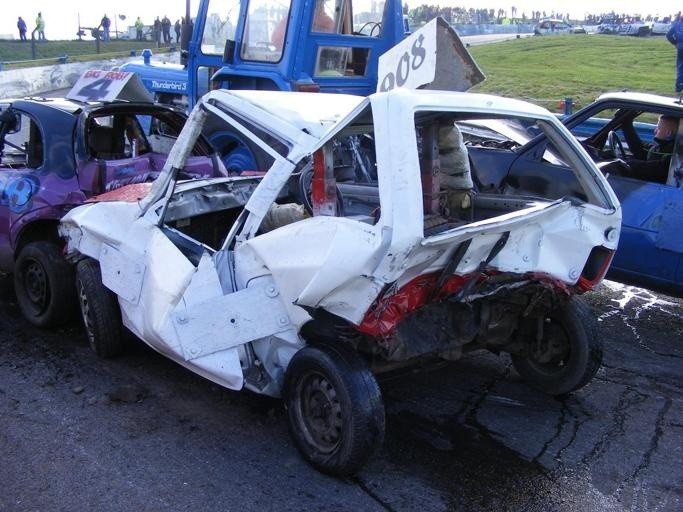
[100,13,110,43]
[134,16,142,43]
[624,114,681,186]
[173,18,179,44]
[16,17,27,41]
[34,12,45,42]
[160,14,172,43]
[665,16,683,94]
[153,15,161,45]
